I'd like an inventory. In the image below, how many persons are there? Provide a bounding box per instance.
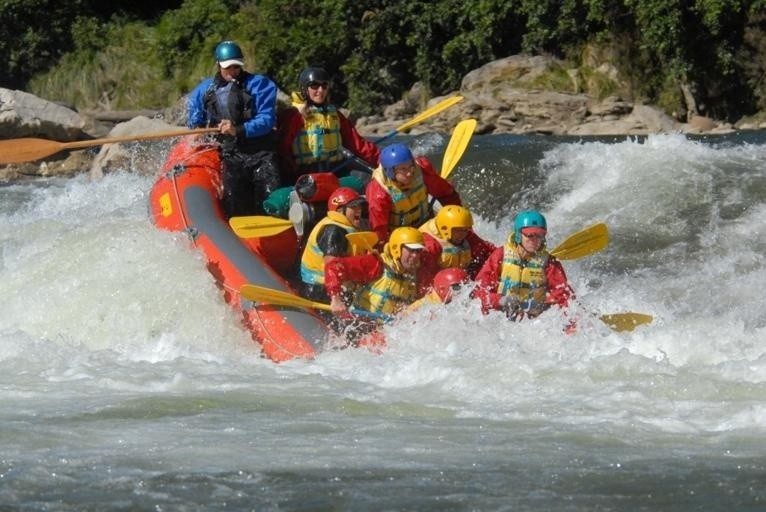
[185,43,283,220]
[416,205,497,302]
[275,68,382,186]
[469,209,588,336]
[365,145,462,253]
[406,268,470,319]
[325,227,429,329]
[299,187,366,337]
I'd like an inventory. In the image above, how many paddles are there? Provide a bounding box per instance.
[551,223,608,259]
[0,128,222,164]
[240,284,397,322]
[428,119,477,212]
[328,96,464,172]
[600,313,653,333]
[229,216,294,239]
[346,232,378,255]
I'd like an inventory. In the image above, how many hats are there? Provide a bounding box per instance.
[216,57,243,68]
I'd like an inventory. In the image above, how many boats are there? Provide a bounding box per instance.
[149,133,577,365]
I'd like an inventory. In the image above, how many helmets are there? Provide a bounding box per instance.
[214,41,243,60]
[327,143,546,303]
[298,67,329,99]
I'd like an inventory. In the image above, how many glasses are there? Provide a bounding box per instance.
[308,82,328,88]
